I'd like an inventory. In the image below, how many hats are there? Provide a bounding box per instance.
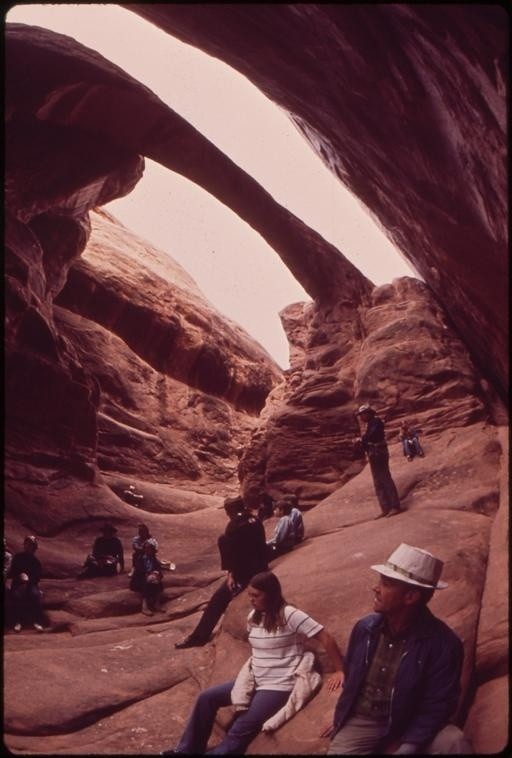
[99,524,118,533]
[355,404,370,415]
[223,494,241,506]
[371,542,449,590]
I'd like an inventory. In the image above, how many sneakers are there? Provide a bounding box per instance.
[14,623,44,632]
[175,638,204,648]
[142,605,167,617]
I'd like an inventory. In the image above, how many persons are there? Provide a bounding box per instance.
[127,491,304,648]
[3,537,12,581]
[352,403,402,521]
[6,535,46,632]
[317,542,474,757]
[398,419,425,462]
[158,570,345,757]
[78,523,124,578]
